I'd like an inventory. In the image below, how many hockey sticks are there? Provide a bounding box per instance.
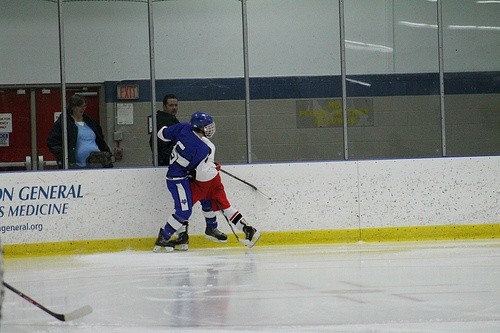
[216,202,252,246]
[2,281,93,322]
[219,169,271,200]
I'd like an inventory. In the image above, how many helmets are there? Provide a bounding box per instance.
[191,111,215,138]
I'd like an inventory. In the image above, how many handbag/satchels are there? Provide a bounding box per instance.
[86,151,113,168]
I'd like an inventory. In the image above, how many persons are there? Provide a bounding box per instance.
[47,94,112,170]
[147,93,180,166]
[168,160,261,251]
[153,111,228,253]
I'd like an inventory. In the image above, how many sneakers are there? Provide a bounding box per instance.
[168,232,189,251]
[204,222,227,244]
[242,226,261,248]
[153,228,175,253]
